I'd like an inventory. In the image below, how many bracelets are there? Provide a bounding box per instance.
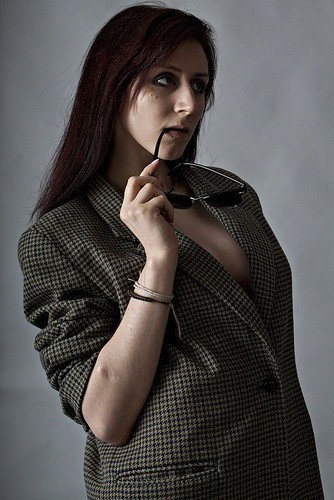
[126,270,174,307]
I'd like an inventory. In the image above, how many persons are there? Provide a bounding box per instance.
[16,3,324,500]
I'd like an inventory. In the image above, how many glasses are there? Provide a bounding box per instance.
[151,128,247,209]
